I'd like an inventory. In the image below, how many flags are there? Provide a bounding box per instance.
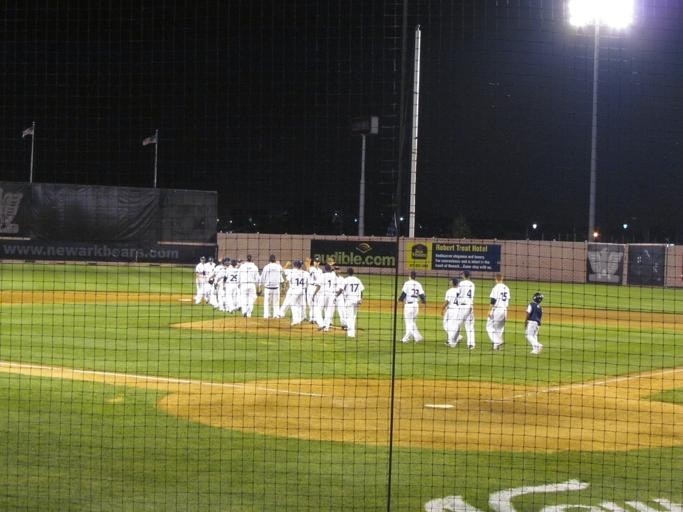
[142,131,157,145]
[22,126,33,137]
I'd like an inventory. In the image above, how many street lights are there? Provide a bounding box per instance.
[565,0,636,241]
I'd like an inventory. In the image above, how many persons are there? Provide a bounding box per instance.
[486,274,510,348]
[444,269,476,349]
[441,277,462,343]
[398,270,426,343]
[523,292,544,353]
[196,254,364,338]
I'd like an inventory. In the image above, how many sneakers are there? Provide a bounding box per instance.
[400,337,504,350]
[531,345,542,354]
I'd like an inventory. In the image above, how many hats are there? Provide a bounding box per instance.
[199,255,242,266]
[410,270,417,277]
[450,270,471,286]
[313,257,353,274]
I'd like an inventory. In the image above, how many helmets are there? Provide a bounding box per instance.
[269,255,276,260]
[532,292,544,303]
[293,259,303,268]
